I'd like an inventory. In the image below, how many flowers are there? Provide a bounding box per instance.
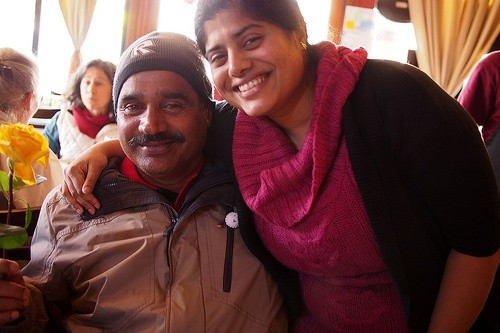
[0,125,49,249]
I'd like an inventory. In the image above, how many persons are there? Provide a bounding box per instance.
[0,31,288,332]
[457,49,500,193]
[60,0,500,333]
[0,47,119,205]
